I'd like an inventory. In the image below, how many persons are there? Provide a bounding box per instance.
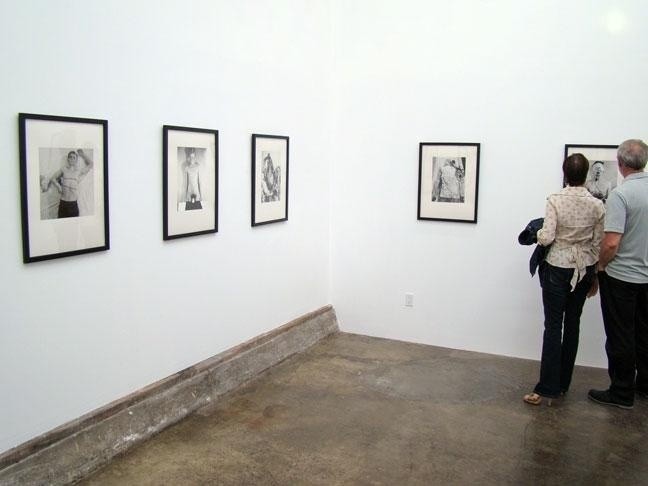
[262,153,281,203]
[40,147,93,220]
[180,147,204,211]
[518,153,604,405]
[587,160,611,201]
[587,138,647,411]
[432,157,465,203]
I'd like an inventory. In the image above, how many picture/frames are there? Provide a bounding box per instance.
[250,133,289,226]
[161,124,218,240]
[562,143,624,204]
[18,112,109,263]
[416,141,479,223]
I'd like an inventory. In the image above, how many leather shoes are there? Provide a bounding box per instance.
[588,389,633,409]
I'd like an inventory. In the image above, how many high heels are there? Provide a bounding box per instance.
[524,393,552,406]
